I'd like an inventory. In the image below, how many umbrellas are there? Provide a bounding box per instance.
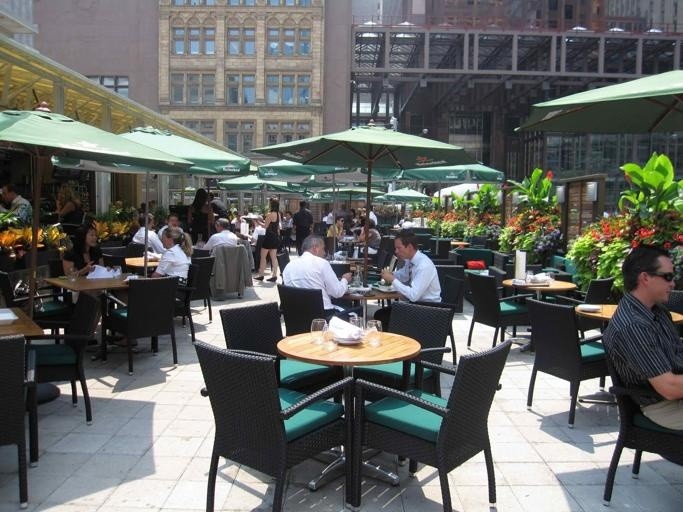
[375,187,432,228]
[309,183,386,235]
[258,158,357,260]
[514,70,682,134]
[251,118,483,328]
[217,173,307,220]
[0,102,195,345]
[51,125,250,277]
[372,164,504,256]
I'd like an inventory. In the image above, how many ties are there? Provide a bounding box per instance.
[407,262,414,285]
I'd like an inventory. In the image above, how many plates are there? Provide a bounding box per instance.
[578,303,600,312]
[367,284,400,293]
[333,338,361,346]
[0,309,19,324]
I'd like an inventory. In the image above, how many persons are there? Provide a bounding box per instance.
[351,209,357,227]
[231,211,246,230]
[63,223,103,345]
[399,217,405,224]
[56,187,81,224]
[374,232,442,332]
[138,200,158,214]
[281,212,294,241]
[204,218,238,256]
[327,216,344,255]
[131,213,167,254]
[402,218,412,229]
[246,215,267,254]
[230,203,237,213]
[325,209,333,230]
[369,206,378,227]
[337,204,352,229]
[188,188,212,245]
[157,215,183,241]
[0,183,34,229]
[207,192,232,223]
[360,219,381,255]
[151,227,193,286]
[254,198,280,282]
[294,201,313,256]
[323,215,328,235]
[231,211,239,225]
[282,235,352,312]
[602,243,683,430]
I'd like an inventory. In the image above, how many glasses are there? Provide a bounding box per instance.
[647,271,675,281]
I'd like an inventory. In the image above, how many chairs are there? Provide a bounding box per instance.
[365,246,390,285]
[429,237,463,258]
[207,245,245,301]
[277,251,290,286]
[0,338,38,510]
[602,329,683,507]
[101,239,129,313]
[2,269,77,347]
[149,264,200,346]
[354,302,452,465]
[23,292,99,427]
[179,254,217,326]
[192,339,353,512]
[276,283,347,340]
[387,274,467,364]
[220,303,343,455]
[352,338,515,512]
[125,242,154,257]
[468,272,532,351]
[248,235,266,272]
[101,276,180,377]
[362,255,398,310]
[524,297,613,428]
[340,236,365,258]
[575,275,618,344]
[329,264,351,286]
[49,259,75,347]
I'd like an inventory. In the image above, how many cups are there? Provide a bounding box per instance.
[311,318,326,345]
[367,320,382,348]
[322,324,338,352]
[350,317,362,328]
[68,268,79,282]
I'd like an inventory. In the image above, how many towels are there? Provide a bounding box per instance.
[328,315,367,341]
